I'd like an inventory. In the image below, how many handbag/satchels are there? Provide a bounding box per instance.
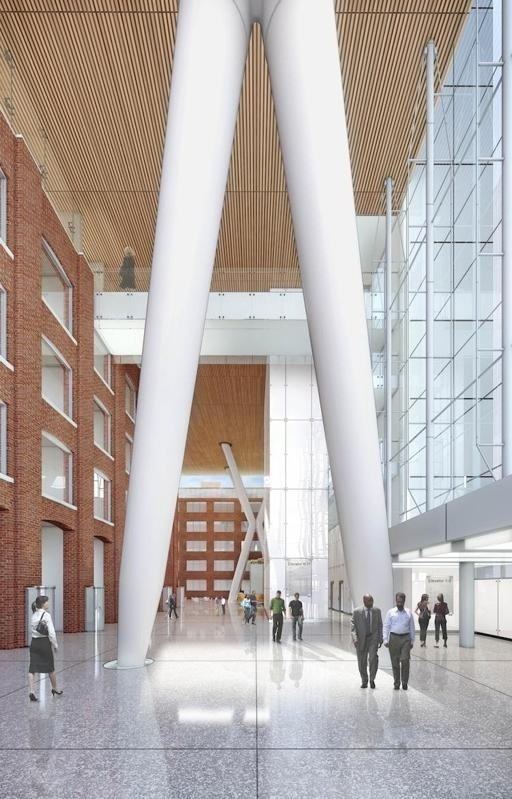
[37,621,48,635]
[423,608,430,618]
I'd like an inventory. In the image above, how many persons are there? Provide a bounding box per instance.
[432,648,448,692]
[415,647,431,690]
[350,593,383,689]
[240,594,251,625]
[382,592,415,691]
[169,593,179,618]
[433,593,449,648]
[385,690,414,754]
[288,640,304,688]
[118,246,136,292]
[28,596,63,703]
[248,590,258,625]
[270,590,287,644]
[270,643,286,690]
[355,687,384,756]
[415,594,431,648]
[221,596,226,615]
[289,593,304,641]
[28,694,62,772]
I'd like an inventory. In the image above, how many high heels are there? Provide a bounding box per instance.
[29,693,38,701]
[52,689,63,696]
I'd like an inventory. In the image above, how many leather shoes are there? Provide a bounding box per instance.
[395,684,399,689]
[361,682,367,687]
[403,684,407,689]
[370,681,375,688]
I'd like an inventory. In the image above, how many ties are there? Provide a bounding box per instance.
[366,610,370,634]
